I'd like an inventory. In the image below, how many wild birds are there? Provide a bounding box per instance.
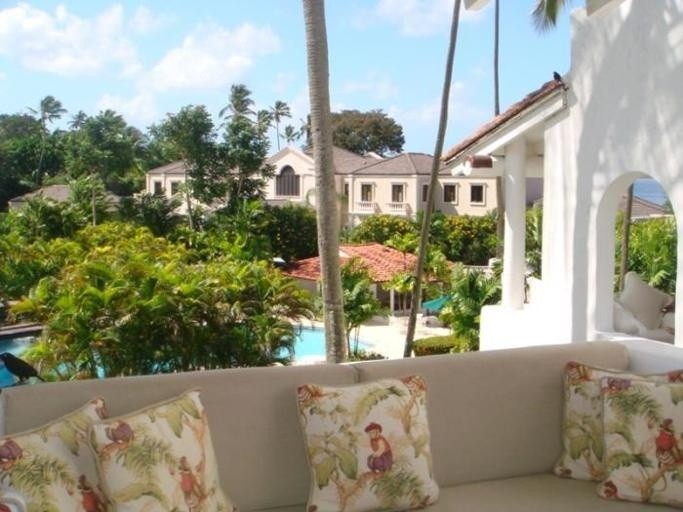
[0,352,46,382]
[552,70,561,83]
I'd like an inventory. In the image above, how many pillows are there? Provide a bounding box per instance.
[296,374,439,512]
[596,376,683,509]
[552,361,627,482]
[87,388,240,512]
[618,271,673,330]
[0,396,109,512]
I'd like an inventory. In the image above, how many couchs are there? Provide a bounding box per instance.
[0,339,683,512]
